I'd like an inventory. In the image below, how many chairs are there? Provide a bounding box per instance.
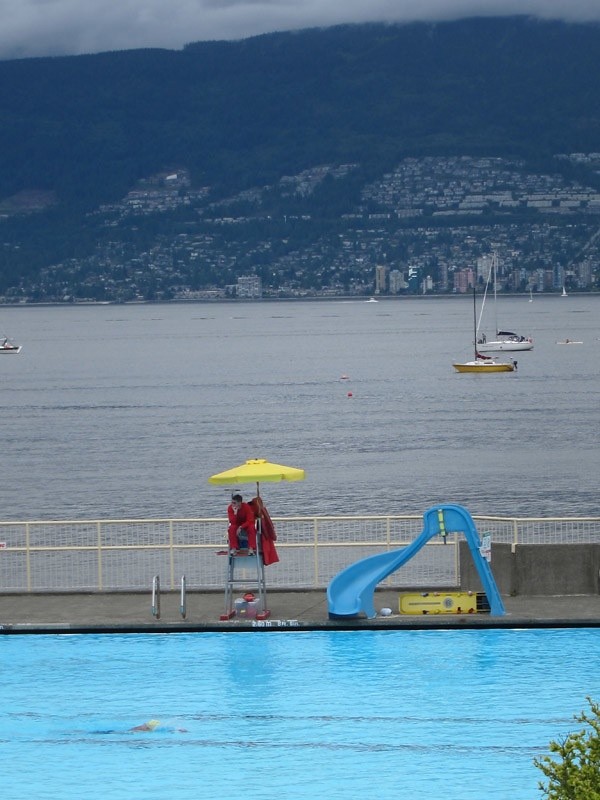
[226,501,261,550]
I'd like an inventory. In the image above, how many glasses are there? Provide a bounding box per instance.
[232,502,236,504]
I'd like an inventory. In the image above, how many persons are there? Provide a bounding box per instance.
[227,495,256,555]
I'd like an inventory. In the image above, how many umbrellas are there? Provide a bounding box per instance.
[208,458,305,496]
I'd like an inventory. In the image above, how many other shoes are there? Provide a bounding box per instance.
[248,550,255,556]
[230,550,237,555]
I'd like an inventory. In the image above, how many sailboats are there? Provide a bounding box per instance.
[451,260,517,372]
[528,287,535,303]
[561,285,569,296]
[476,252,536,352]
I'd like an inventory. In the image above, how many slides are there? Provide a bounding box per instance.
[327,532,430,620]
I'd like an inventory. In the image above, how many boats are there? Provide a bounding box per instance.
[0,329,23,354]
[555,341,583,346]
[366,296,378,303]
